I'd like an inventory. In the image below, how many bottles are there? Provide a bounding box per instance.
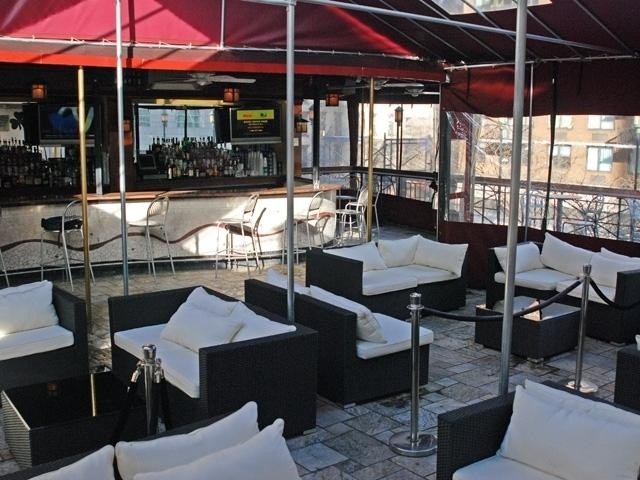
[151,135,247,180]
[0,135,78,191]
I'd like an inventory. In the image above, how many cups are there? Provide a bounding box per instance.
[246,150,277,177]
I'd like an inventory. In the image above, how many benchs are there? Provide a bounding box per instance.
[304,229,472,323]
[613,338,640,413]
[1,398,304,479]
[436,378,640,480]
[243,265,436,410]
[0,279,90,409]
[487,241,640,342]
[106,282,323,442]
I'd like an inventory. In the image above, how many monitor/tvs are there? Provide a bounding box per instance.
[228,107,283,144]
[138,154,156,170]
[23,104,39,145]
[38,104,98,144]
[214,109,230,143]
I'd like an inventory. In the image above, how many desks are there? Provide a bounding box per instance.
[472,295,584,361]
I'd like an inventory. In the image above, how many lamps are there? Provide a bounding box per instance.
[291,111,309,133]
[220,85,242,104]
[28,81,47,102]
[324,83,342,108]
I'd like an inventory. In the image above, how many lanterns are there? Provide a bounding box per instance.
[223,88,240,101]
[324,92,339,107]
[31,83,47,100]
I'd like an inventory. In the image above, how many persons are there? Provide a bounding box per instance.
[47,106,77,134]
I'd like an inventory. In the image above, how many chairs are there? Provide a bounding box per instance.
[38,198,97,292]
[211,193,264,278]
[282,163,385,266]
[125,195,178,284]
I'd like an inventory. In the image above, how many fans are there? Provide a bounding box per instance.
[153,71,259,89]
[330,76,425,94]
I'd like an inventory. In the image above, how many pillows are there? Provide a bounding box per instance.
[132,417,302,480]
[28,442,116,480]
[376,233,419,267]
[114,399,261,479]
[589,248,640,287]
[306,283,391,347]
[321,239,389,271]
[159,301,245,353]
[224,299,299,345]
[267,267,309,296]
[184,286,239,321]
[413,232,471,278]
[492,242,545,274]
[0,279,60,338]
[539,232,594,276]
[501,383,640,479]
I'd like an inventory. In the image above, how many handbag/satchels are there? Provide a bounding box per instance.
[41,216,83,231]
